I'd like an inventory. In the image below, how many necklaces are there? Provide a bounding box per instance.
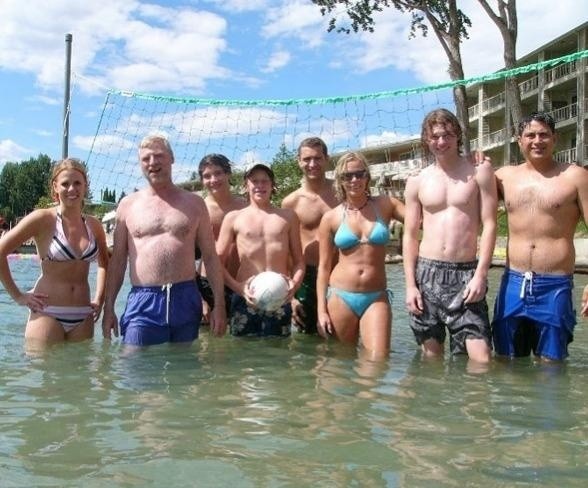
[342,195,370,212]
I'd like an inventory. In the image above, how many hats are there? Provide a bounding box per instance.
[243,160,274,181]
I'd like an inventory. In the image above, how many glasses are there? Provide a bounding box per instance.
[339,169,366,180]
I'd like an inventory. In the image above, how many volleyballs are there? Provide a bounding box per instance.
[249,271,289,311]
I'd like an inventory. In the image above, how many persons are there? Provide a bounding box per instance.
[401,107,499,365]
[195,154,250,328]
[0,159,109,345]
[216,162,304,342]
[281,135,348,335]
[101,135,228,345]
[467,109,588,362]
[316,151,406,361]
